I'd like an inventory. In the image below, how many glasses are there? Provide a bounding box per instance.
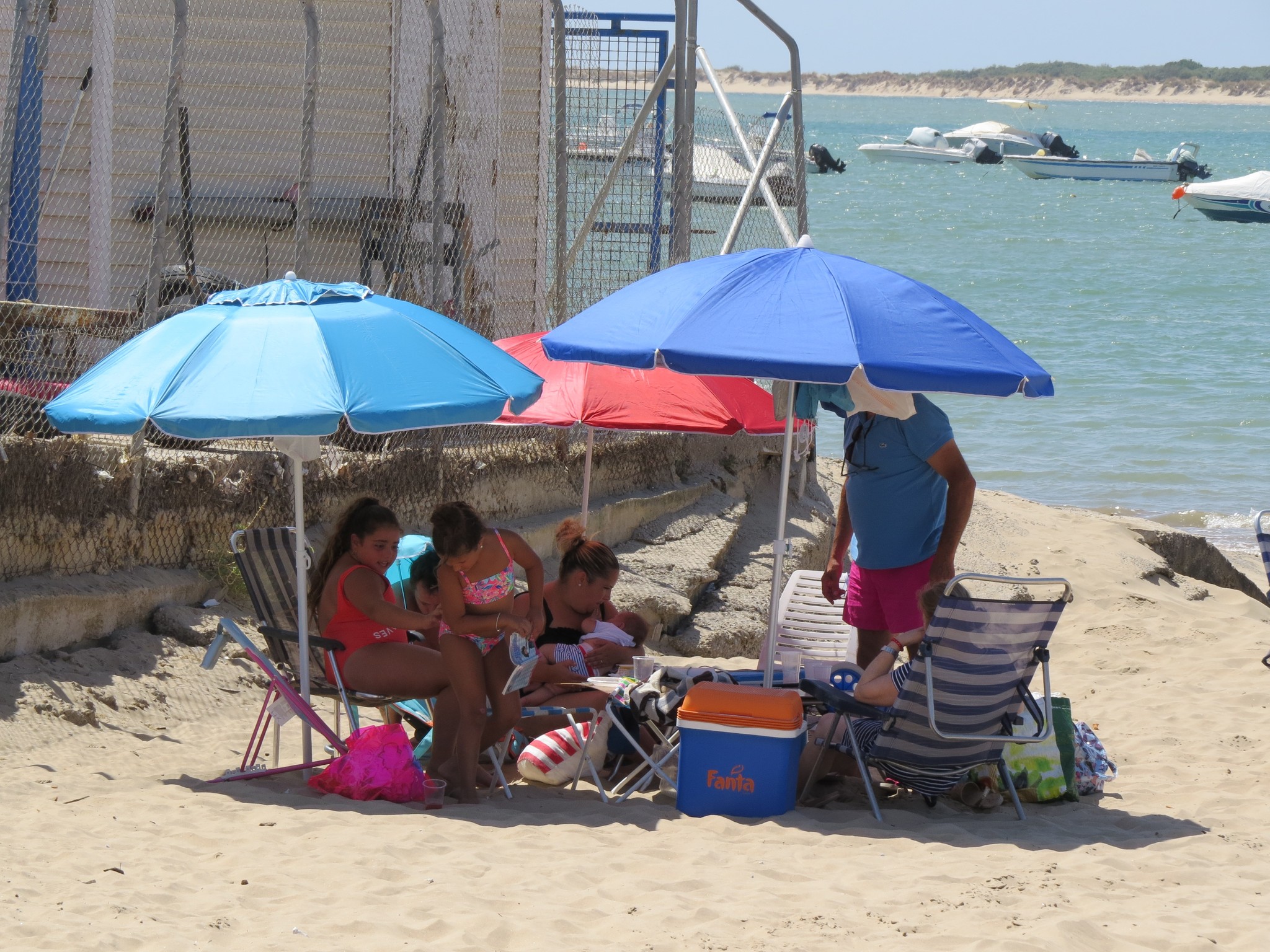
[840,414,876,467]
[840,450,879,477]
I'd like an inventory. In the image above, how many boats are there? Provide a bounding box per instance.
[565,142,672,162]
[1181,170,1270,215]
[647,142,778,203]
[856,98,1048,164]
[1002,141,1200,182]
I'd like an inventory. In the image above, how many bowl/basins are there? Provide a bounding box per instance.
[616,664,634,678]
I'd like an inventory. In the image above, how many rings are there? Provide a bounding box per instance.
[572,684,574,687]
[601,660,603,663]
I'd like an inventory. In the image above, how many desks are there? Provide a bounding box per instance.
[606,678,679,804]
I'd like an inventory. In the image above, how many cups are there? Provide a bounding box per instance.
[813,665,832,685]
[423,779,447,810]
[804,658,823,680]
[780,651,802,684]
[632,656,655,682]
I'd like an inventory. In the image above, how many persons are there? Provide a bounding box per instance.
[786,580,1025,799]
[377,548,441,726]
[520,608,649,708]
[519,517,646,740]
[314,497,504,800]
[428,495,546,803]
[796,382,977,675]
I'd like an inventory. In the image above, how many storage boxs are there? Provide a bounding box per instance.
[675,681,810,817]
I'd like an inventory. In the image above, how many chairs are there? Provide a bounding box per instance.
[230,524,1074,822]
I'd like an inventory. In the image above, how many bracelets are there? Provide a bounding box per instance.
[496,612,502,632]
[891,637,904,652]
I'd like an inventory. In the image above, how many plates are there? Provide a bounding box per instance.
[587,677,622,687]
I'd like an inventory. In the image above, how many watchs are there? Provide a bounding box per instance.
[880,644,899,661]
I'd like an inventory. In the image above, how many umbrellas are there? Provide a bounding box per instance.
[42,269,546,792]
[534,235,1060,684]
[486,331,812,541]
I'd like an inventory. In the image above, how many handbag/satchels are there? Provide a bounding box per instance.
[966,691,1081,803]
[1074,722,1118,796]
[308,724,438,802]
